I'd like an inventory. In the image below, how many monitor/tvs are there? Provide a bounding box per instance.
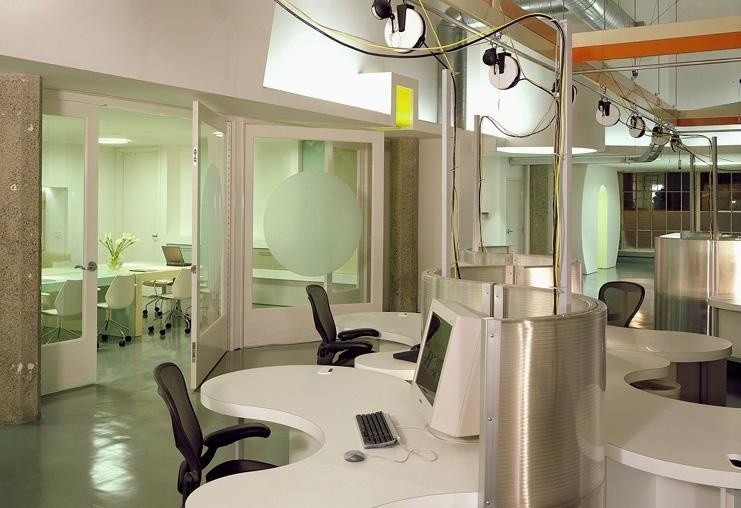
[410,296,490,445]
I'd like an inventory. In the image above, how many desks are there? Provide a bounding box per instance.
[183,357,486,508]
[333,309,437,382]
[599,344,741,507]
[605,320,735,407]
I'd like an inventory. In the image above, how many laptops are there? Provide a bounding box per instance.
[161,245,192,267]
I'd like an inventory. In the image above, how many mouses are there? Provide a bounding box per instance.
[343,450,365,462]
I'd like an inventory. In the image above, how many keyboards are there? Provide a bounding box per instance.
[355,409,401,449]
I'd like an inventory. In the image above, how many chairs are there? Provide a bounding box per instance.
[152,361,281,507]
[599,278,647,331]
[42,250,213,351]
[306,283,384,368]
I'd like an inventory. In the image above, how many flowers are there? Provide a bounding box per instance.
[97,228,143,265]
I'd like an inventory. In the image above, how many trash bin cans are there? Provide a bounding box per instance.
[629,378,681,403]
